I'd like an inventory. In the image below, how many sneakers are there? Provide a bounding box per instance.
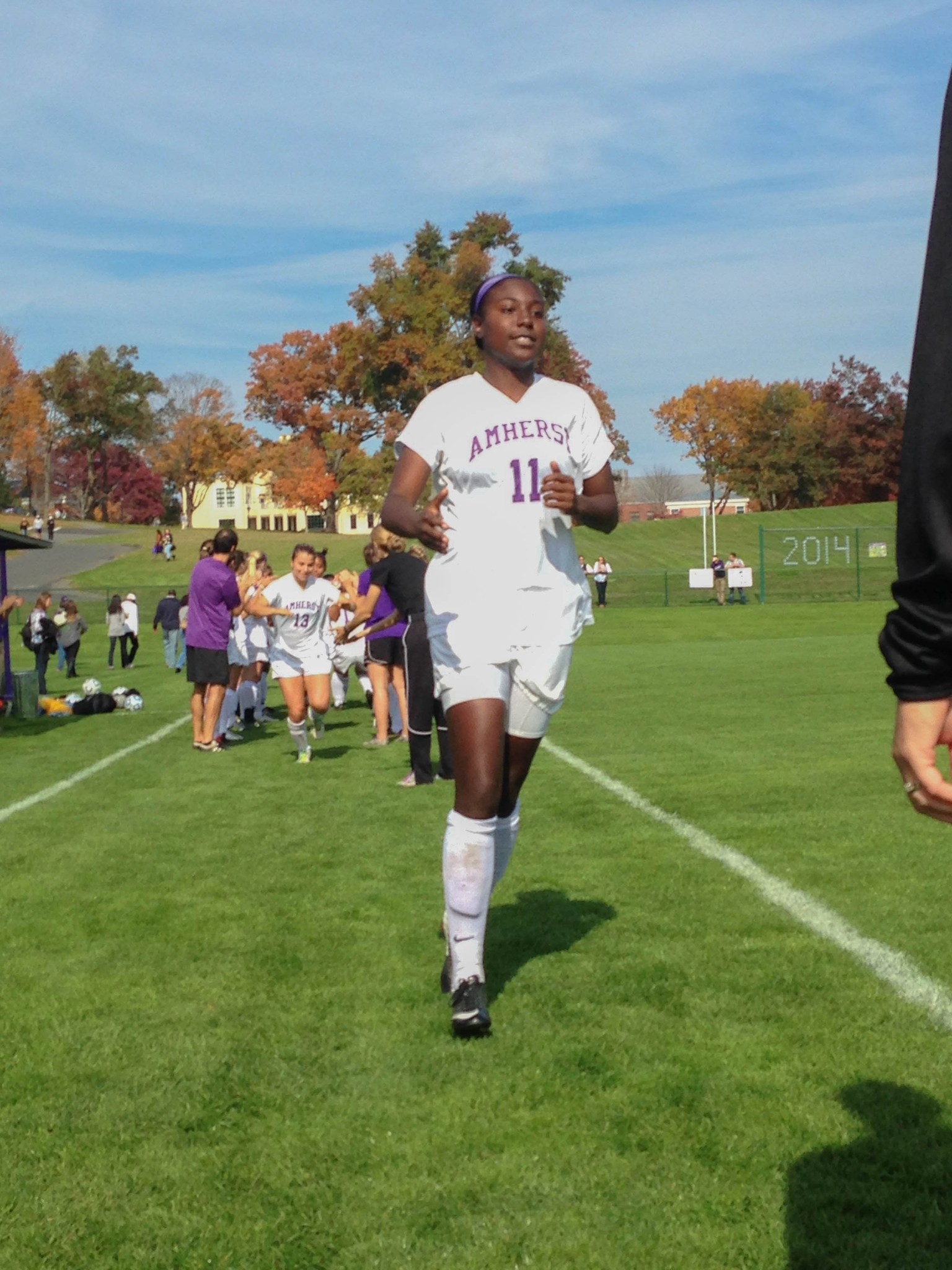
[440,945,451,992]
[199,741,227,752]
[298,746,312,764]
[449,980,491,1029]
[308,707,325,740]
[192,743,201,749]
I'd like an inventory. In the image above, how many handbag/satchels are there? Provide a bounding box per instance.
[73,693,116,714]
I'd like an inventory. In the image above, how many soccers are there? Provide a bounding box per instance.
[125,693,144,711]
[111,686,129,709]
[63,691,84,709]
[82,678,102,696]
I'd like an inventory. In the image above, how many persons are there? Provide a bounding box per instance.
[873,72,952,819]
[0,594,24,719]
[19,516,29,536]
[46,512,54,540]
[377,271,624,1034]
[725,552,747,605]
[17,522,453,787]
[578,555,593,576]
[593,556,613,608]
[710,555,726,606]
[33,514,43,540]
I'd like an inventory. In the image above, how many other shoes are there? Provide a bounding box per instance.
[216,709,279,746]
[366,737,389,745]
[395,773,416,786]
[366,691,373,708]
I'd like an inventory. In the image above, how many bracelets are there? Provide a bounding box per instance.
[344,626,350,634]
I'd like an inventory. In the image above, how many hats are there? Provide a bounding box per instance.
[127,593,136,600]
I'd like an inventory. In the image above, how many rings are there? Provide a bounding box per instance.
[903,777,922,794]
